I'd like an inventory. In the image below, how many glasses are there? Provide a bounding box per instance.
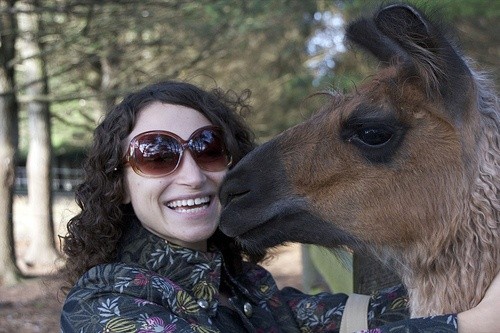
[121,126,233,177]
